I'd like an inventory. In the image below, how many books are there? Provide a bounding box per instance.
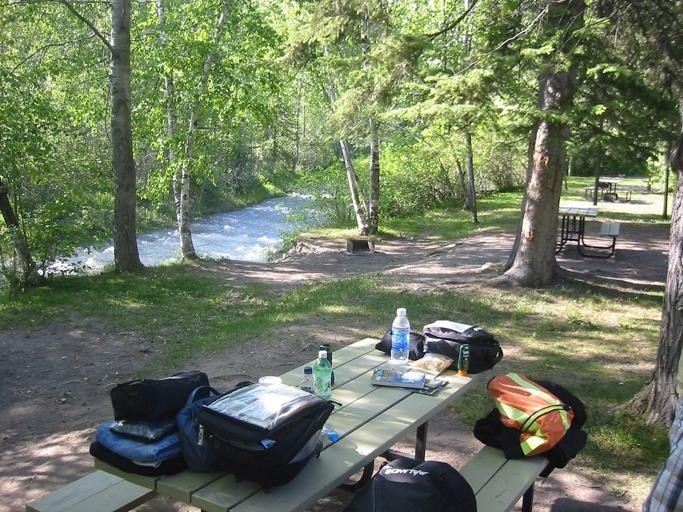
[405,378,449,396]
[369,368,426,390]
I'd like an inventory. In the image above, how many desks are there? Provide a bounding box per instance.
[554,203,600,257]
[95,337,472,511]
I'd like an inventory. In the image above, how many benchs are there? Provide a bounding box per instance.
[25,469,159,512]
[458,444,551,512]
[597,222,620,258]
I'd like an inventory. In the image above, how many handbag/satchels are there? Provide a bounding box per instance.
[375,319,503,374]
[474,380,587,469]
[110,370,210,424]
[343,458,477,512]
[176,375,342,494]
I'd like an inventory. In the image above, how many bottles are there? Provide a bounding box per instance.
[389,307,410,365]
[299,367,316,395]
[320,421,338,441]
[457,344,471,377]
[319,343,334,386]
[314,349,332,401]
